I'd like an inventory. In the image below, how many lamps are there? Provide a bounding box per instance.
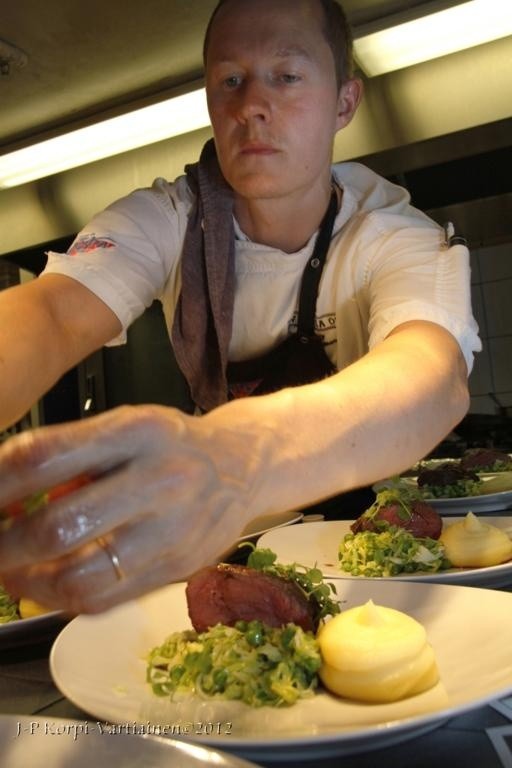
[0,0,512,190]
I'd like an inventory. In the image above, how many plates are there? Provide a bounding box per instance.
[255,515,512,589]
[232,511,304,552]
[374,453,512,515]
[0,590,78,637]
[0,710,267,768]
[48,576,512,761]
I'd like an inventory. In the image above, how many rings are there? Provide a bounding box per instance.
[94,535,128,586]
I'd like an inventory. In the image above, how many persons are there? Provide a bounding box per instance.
[2,2,480,614]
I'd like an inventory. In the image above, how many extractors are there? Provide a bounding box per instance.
[338,117,512,252]
